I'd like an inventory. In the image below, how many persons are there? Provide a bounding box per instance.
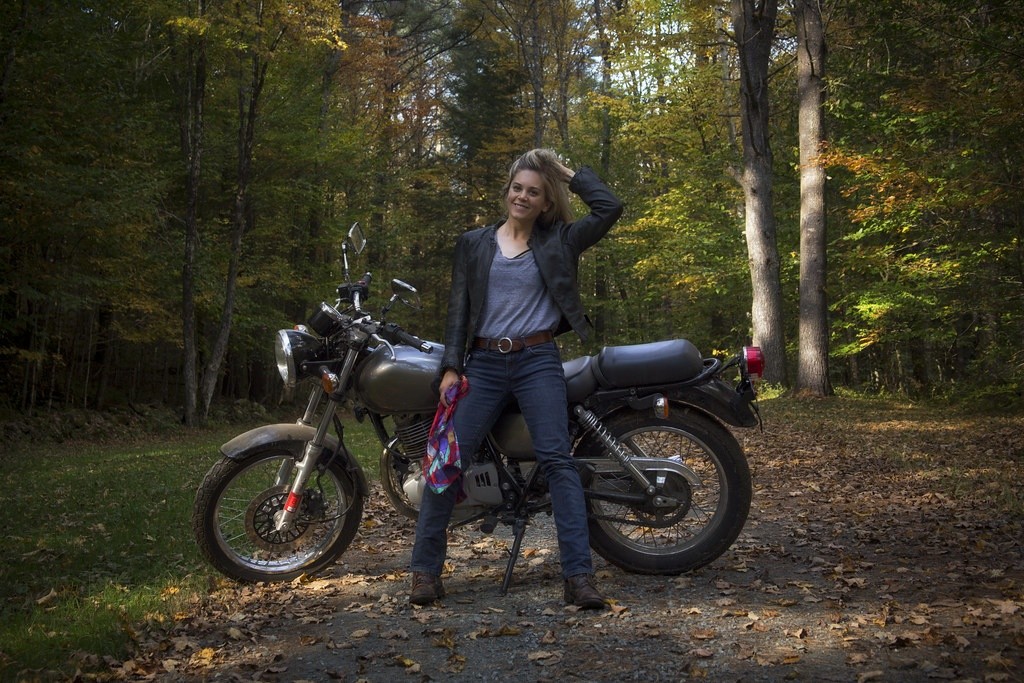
[410,149,624,610]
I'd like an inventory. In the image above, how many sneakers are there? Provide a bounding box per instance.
[409,572,445,604]
[565,573,604,609]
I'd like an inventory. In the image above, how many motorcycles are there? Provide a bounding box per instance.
[190,221,766,598]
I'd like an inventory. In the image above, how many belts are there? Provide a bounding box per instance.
[473,330,553,354]
